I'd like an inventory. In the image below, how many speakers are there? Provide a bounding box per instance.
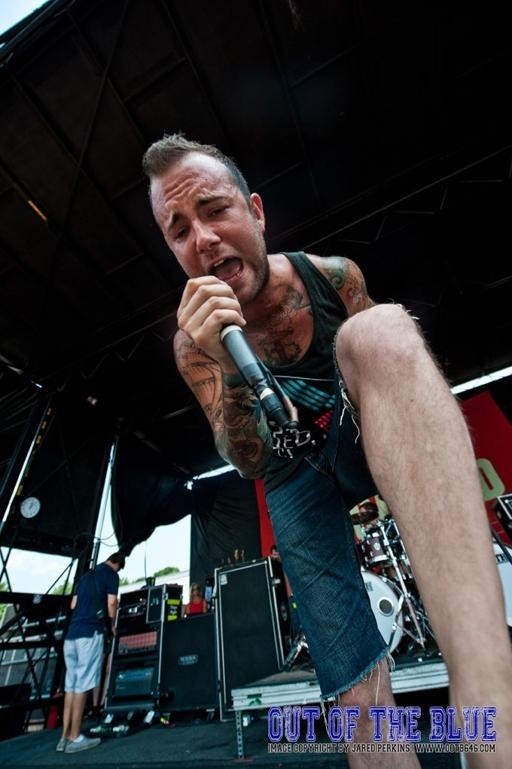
[159,612,219,711]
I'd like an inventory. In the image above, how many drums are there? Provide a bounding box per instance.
[486,540,512,629]
[359,569,413,665]
[363,531,398,567]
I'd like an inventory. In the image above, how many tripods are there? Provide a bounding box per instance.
[376,521,444,656]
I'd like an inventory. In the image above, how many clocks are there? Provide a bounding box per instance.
[18,496,43,520]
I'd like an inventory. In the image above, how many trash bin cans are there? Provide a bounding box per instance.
[0,682,33,739]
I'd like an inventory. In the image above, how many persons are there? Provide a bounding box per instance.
[143,134,510,768]
[55,553,127,754]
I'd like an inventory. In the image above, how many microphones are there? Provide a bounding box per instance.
[88,538,100,575]
[220,321,290,427]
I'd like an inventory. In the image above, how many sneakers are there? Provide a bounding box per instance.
[55,735,68,753]
[62,734,102,754]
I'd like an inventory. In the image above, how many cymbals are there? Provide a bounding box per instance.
[348,511,378,526]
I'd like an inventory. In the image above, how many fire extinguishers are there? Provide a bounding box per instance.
[46,686,62,730]
